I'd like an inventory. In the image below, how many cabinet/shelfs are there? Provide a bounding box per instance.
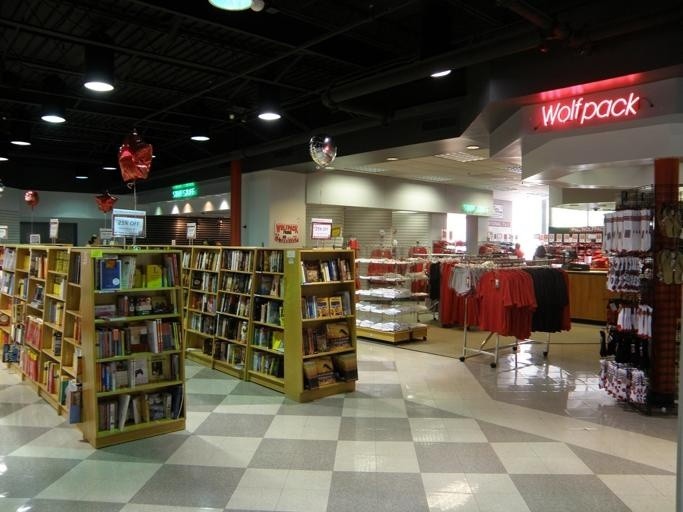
[358,254,429,345]
[101,242,359,404]
[1,243,187,450]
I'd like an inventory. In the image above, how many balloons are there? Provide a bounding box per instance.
[309,135,337,168]
[95,189,121,214]
[24,190,39,208]
[118,142,153,182]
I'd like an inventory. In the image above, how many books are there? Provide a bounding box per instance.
[0,249,83,424]
[303,259,358,390]
[183,251,285,379]
[94,253,184,431]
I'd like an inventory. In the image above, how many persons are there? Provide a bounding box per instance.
[533,245,548,260]
[514,243,523,258]
[88,234,98,245]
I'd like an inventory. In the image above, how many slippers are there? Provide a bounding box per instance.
[657,204,683,285]
